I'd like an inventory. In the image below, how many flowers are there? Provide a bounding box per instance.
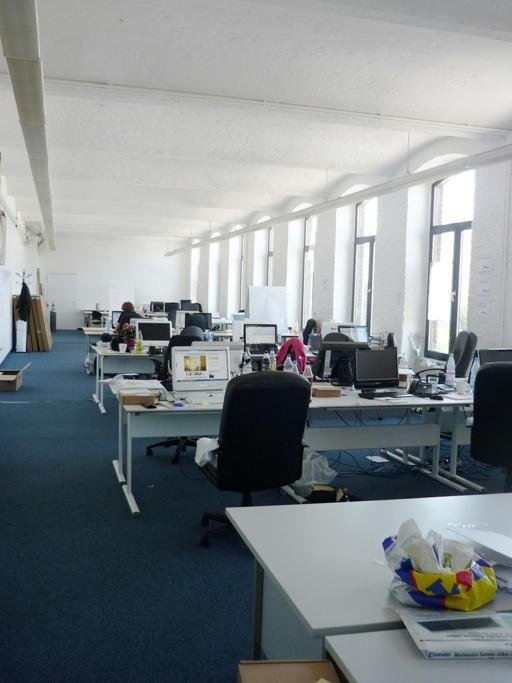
[117,323,136,339]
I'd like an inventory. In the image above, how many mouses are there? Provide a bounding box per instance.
[430,395,444,401]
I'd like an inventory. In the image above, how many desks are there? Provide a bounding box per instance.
[213,330,233,341]
[82,327,117,353]
[325,628,512,682]
[113,386,469,518]
[226,493,512,660]
[91,346,165,415]
[396,377,485,493]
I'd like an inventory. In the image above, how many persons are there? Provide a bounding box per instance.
[116,301,138,331]
[303,318,316,346]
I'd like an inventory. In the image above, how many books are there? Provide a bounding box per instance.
[113,379,165,406]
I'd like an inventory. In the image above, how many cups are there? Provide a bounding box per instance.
[455,380,468,394]
[119,343,127,353]
[427,376,438,394]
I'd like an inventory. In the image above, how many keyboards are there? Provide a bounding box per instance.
[357,390,399,400]
[185,394,224,405]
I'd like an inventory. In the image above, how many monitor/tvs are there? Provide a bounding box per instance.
[243,323,277,354]
[170,345,230,392]
[185,313,212,332]
[338,325,368,343]
[136,321,173,347]
[111,311,122,328]
[312,341,369,380]
[354,347,400,390]
[174,309,200,329]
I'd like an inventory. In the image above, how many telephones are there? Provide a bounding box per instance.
[408,378,443,400]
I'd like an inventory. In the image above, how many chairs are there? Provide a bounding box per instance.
[185,312,212,330]
[183,303,198,312]
[142,336,205,463]
[415,332,478,378]
[465,360,512,490]
[196,371,311,547]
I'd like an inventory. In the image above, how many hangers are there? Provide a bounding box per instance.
[16,271,32,286]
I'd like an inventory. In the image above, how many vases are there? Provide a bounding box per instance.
[126,339,135,352]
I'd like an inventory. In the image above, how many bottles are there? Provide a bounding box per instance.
[242,347,253,374]
[202,330,211,340]
[135,331,144,353]
[283,355,292,373]
[445,353,456,388]
[269,348,276,371]
[398,353,409,388]
[470,358,480,395]
[293,357,299,373]
[261,349,270,370]
[302,361,312,402]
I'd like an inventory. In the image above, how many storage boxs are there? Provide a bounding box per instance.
[237,662,337,683]
[0,369,21,392]
